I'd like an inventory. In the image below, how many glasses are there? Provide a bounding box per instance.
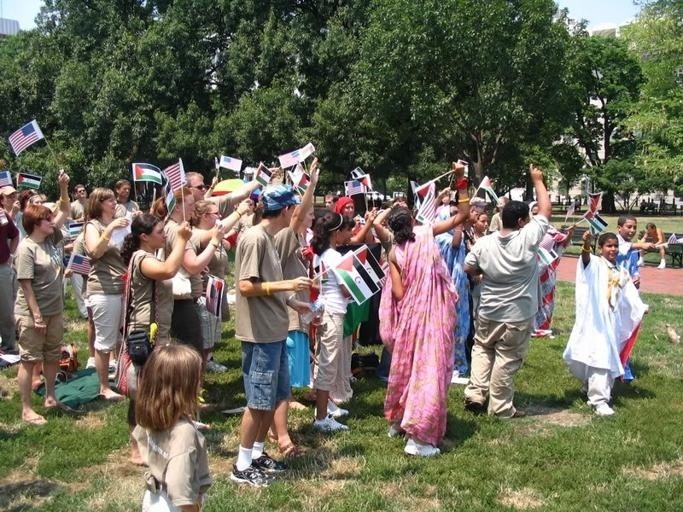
[77,190,85,193]
[190,184,205,190]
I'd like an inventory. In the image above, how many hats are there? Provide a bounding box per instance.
[335,197,352,214]
[262,184,301,210]
[0,186,19,196]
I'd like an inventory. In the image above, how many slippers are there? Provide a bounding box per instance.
[22,415,47,425]
[101,390,126,402]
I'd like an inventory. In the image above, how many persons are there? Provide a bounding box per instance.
[468,212,492,316]
[490,197,513,235]
[132,345,210,512]
[122,214,190,462]
[70,184,91,222]
[111,181,138,219]
[564,230,646,415]
[162,189,254,363]
[469,197,487,220]
[529,201,568,338]
[325,192,339,213]
[182,171,207,201]
[573,199,581,212]
[639,197,678,213]
[275,160,311,460]
[311,213,350,433]
[234,183,309,487]
[386,162,469,455]
[85,189,132,400]
[437,191,459,222]
[16,171,70,425]
[0,222,22,366]
[463,162,553,417]
[615,216,658,385]
[636,223,668,268]
[335,197,377,244]
[1,186,24,225]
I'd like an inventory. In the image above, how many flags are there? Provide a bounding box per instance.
[7,120,42,156]
[255,162,270,185]
[413,182,435,223]
[164,160,186,193]
[352,246,385,286]
[351,168,372,187]
[133,164,161,183]
[586,213,605,231]
[479,177,499,203]
[15,174,43,190]
[343,179,366,194]
[204,276,225,316]
[1,171,11,185]
[278,143,314,168]
[587,194,602,210]
[329,252,379,304]
[667,233,682,247]
[217,156,240,171]
[65,254,91,276]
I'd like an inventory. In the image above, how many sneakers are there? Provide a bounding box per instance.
[592,403,615,416]
[637,261,645,267]
[657,264,666,270]
[252,452,288,472]
[314,399,349,418]
[404,438,441,457]
[207,361,228,373]
[108,359,118,368]
[463,397,483,410]
[313,415,349,431]
[514,410,525,418]
[229,465,275,488]
[86,355,97,371]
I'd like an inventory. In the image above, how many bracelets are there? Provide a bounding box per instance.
[264,281,273,294]
[209,241,218,246]
[581,244,593,252]
[59,196,71,204]
[457,198,468,203]
[100,231,110,241]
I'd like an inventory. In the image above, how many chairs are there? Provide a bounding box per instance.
[559,225,596,255]
[638,231,683,268]
[643,204,658,215]
[549,202,574,217]
[659,204,677,215]
